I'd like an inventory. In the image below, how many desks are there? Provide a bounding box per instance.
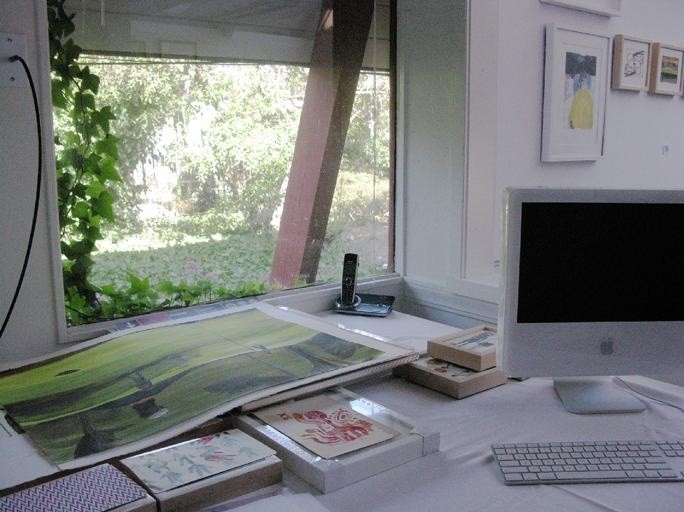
[0,311,683,512]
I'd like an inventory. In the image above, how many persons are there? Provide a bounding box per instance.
[573,56,592,94]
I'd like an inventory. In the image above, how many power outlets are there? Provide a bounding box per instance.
[1,33,30,88]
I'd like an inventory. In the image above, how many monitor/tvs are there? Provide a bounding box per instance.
[495,187,684,414]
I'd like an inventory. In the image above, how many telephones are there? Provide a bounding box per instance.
[335,253,361,310]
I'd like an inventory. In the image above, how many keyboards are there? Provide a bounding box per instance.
[490,439,684,485]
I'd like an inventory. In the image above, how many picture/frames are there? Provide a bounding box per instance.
[650,42,684,97]
[540,22,614,163]
[427,325,497,372]
[408,354,506,400]
[540,0,624,17]
[236,386,439,494]
[610,34,653,92]
